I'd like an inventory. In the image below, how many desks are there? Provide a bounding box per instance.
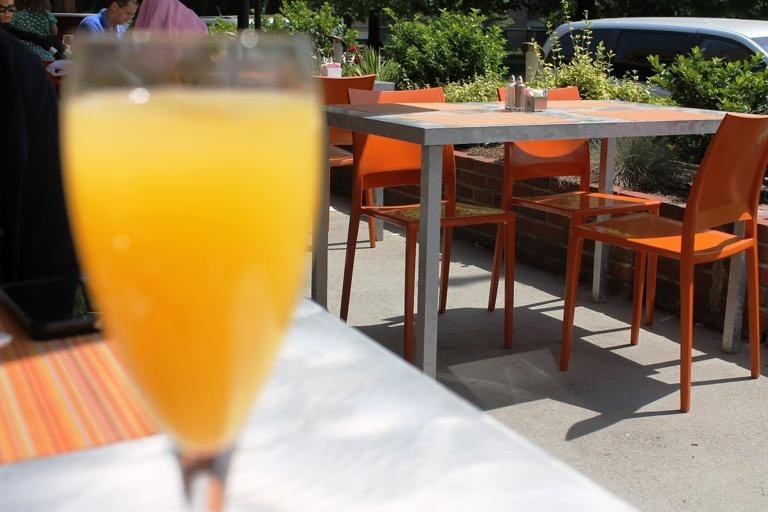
[317,100,768,416]
[2,296,641,511]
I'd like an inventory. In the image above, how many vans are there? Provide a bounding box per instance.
[536,16,768,100]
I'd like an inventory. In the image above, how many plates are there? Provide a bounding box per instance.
[45,59,71,76]
[59,28,326,511]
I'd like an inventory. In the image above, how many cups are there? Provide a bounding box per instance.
[327,67,342,76]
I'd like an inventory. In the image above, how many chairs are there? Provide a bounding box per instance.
[559,111,768,411]
[489,77,664,331]
[340,81,520,377]
[310,73,379,248]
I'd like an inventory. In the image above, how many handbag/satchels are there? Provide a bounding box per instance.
[0,23,100,340]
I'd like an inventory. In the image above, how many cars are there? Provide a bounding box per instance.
[200,13,293,25]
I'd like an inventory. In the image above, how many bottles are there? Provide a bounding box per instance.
[514,74,525,111]
[505,73,514,110]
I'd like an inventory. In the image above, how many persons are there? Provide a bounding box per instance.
[9,1,59,62]
[72,0,139,44]
[1,0,14,24]
